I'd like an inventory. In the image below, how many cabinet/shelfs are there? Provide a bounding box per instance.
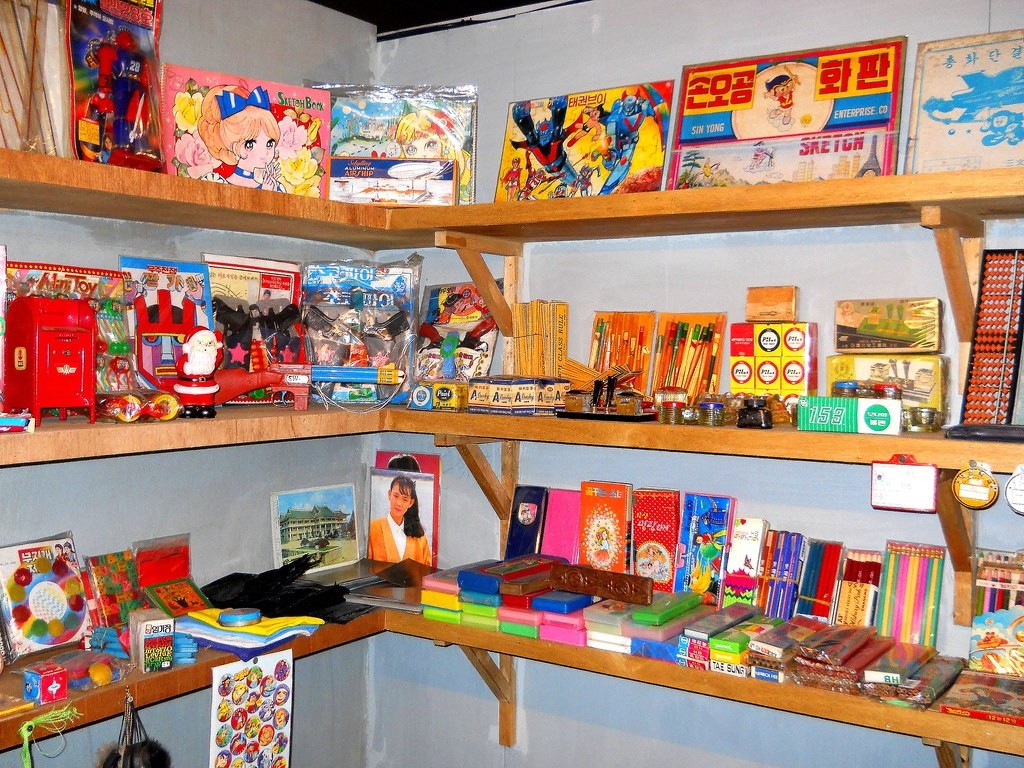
[386,169,1024,768]
[1,148,386,768]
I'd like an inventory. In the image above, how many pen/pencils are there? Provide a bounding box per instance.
[796,538,947,648]
[747,615,967,712]
[976,552,1024,616]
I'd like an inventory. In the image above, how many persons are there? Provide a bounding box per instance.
[367,474,433,568]
[173,326,224,418]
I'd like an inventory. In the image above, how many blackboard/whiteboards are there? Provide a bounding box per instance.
[870,454,937,513]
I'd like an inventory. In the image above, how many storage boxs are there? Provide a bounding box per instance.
[721,522,770,607]
[128,609,174,675]
[672,491,736,606]
[433,381,468,409]
[751,667,784,684]
[730,321,818,398]
[756,530,807,622]
[469,378,570,416]
[541,489,581,565]
[675,603,785,678]
[827,355,949,425]
[747,286,799,323]
[864,643,938,686]
[939,670,1024,727]
[504,486,549,561]
[798,396,903,435]
[749,615,829,658]
[834,298,942,353]
[24,663,68,705]
[631,488,679,593]
[420,553,718,663]
[324,157,460,209]
[579,481,633,575]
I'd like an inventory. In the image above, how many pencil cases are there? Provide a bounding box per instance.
[419,553,708,669]
[502,480,738,612]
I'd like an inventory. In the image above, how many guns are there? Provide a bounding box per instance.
[302,304,412,343]
[418,316,497,353]
[211,296,304,337]
[212,364,405,413]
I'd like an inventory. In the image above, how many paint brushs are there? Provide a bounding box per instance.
[588,312,723,407]
